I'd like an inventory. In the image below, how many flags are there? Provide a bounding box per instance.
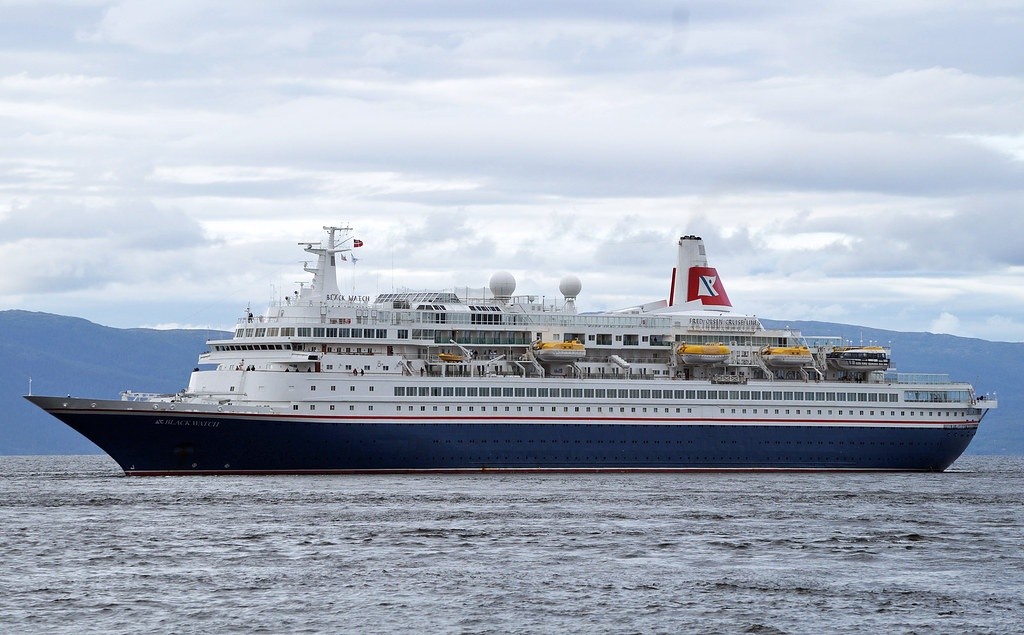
[354,239,363,247]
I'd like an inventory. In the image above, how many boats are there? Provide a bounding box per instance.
[826,345,890,372]
[533,340,587,363]
[438,352,471,363]
[676,340,731,364]
[17,223,1003,474]
[757,344,813,368]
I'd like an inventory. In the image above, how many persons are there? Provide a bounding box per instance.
[285,367,311,372]
[236,365,255,371]
[352,368,364,376]
[420,365,428,377]
[248,312,253,323]
[855,375,862,383]
